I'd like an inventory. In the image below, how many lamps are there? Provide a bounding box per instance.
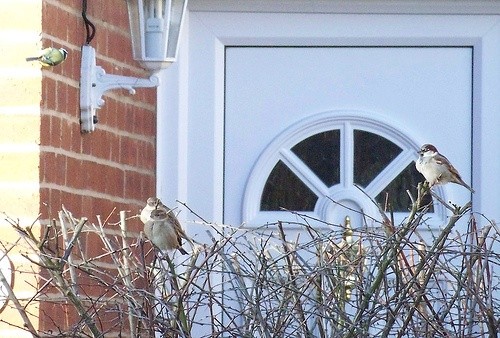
[80,0,189,136]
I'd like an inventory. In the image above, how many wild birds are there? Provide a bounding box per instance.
[141,197,189,257]
[416,144,477,197]
[25,47,69,71]
